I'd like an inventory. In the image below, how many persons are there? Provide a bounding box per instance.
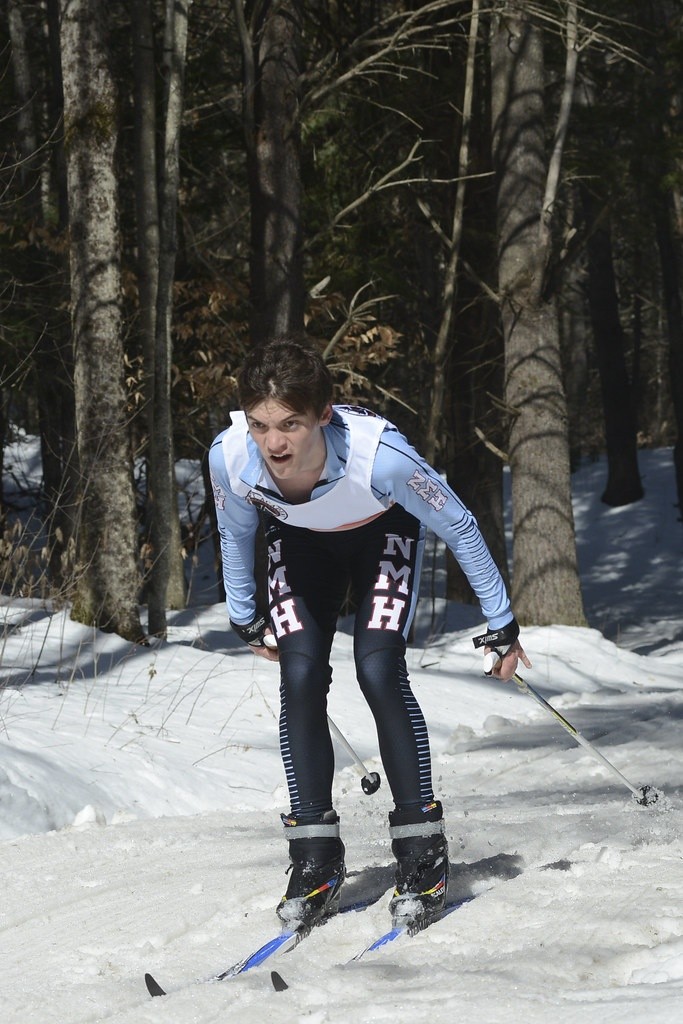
[206,345,533,922]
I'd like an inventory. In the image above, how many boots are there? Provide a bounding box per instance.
[387,802,451,918]
[276,811,346,927]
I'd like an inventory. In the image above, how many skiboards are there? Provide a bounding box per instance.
[144,887,503,999]
